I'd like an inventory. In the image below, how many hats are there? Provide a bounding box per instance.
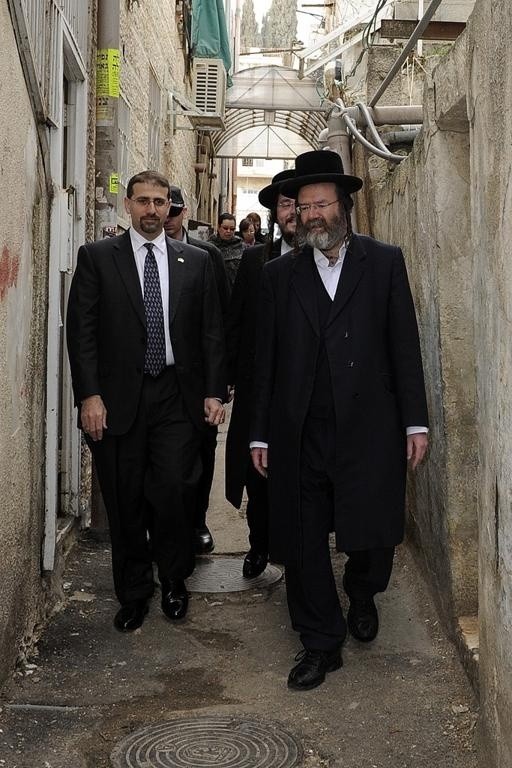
[259,169,297,208]
[278,150,363,201]
[167,185,185,208]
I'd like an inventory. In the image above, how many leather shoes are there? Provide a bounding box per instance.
[346,592,379,644]
[195,526,215,555]
[287,645,342,691]
[114,593,147,632]
[242,545,267,579]
[161,585,189,622]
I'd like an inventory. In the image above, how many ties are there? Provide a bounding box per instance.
[141,243,168,380]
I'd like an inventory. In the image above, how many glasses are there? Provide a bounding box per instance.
[167,206,183,217]
[128,197,169,206]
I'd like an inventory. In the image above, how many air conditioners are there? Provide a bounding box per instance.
[187,56,229,131]
